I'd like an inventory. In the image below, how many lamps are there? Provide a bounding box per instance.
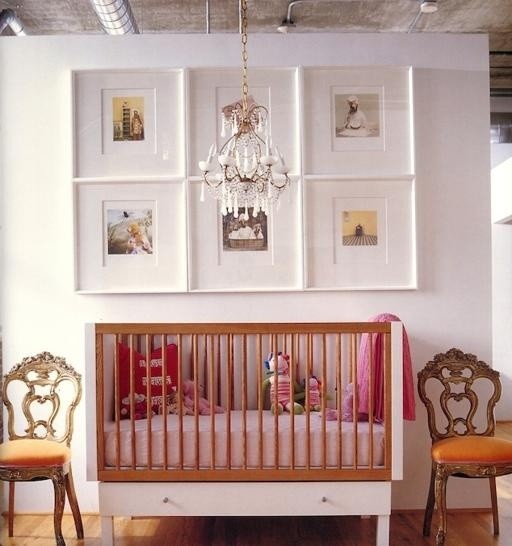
[196,0,292,222]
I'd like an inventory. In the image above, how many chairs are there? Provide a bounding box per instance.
[1,349,86,546]
[416,346,512,545]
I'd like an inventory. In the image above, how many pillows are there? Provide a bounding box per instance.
[111,341,180,422]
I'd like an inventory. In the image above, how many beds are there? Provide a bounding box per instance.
[82,318,406,546]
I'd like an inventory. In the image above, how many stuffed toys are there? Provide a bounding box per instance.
[262,351,304,415]
[300,376,335,412]
[182,378,224,416]
[120,392,154,420]
[169,390,194,416]
[320,383,360,423]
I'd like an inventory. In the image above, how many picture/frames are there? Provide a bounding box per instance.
[302,65,415,176]
[187,176,302,293]
[185,65,301,176]
[68,180,186,297]
[302,178,416,292]
[70,67,185,179]
